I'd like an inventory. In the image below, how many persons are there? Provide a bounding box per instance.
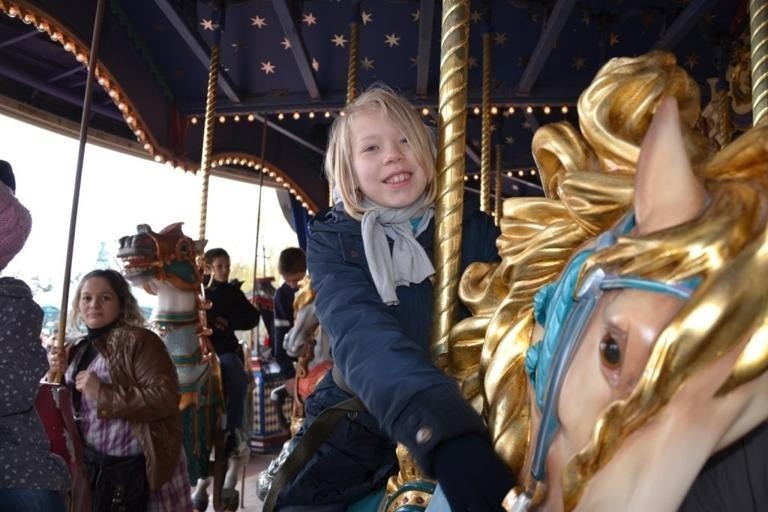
[39,322,74,385]
[272,246,307,399]
[201,246,262,460]
[46,268,195,511]
[258,82,521,512]
[0,158,73,512]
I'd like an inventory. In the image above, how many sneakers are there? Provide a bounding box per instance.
[230,425,251,459]
